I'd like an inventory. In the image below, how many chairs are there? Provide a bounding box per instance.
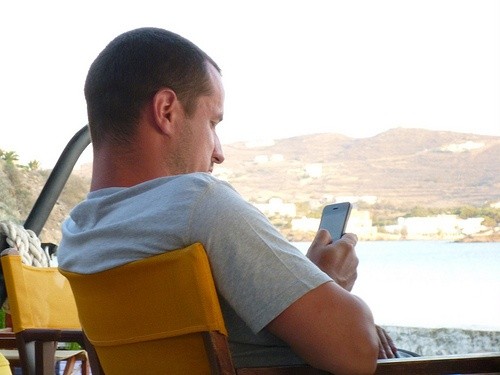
[58,242,500,375]
[0,248,106,375]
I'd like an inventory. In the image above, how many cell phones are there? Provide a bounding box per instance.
[317,202,353,245]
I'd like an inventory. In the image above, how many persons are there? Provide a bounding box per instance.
[55,28,380,375]
